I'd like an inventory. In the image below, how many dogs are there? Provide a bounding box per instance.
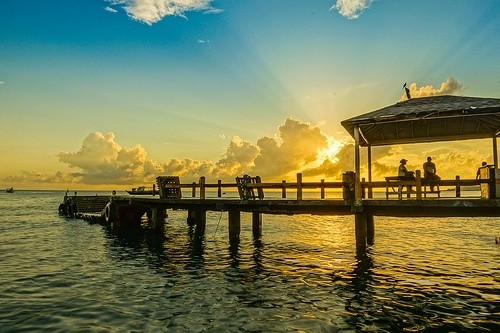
[405,171,415,190]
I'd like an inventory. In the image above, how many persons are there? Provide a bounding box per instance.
[423,157,442,192]
[397,158,415,193]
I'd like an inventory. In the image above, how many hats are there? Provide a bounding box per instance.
[399,159,408,163]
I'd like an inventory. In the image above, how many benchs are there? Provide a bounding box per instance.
[384,176,441,198]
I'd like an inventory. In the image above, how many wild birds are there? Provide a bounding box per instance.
[402,83,406,89]
